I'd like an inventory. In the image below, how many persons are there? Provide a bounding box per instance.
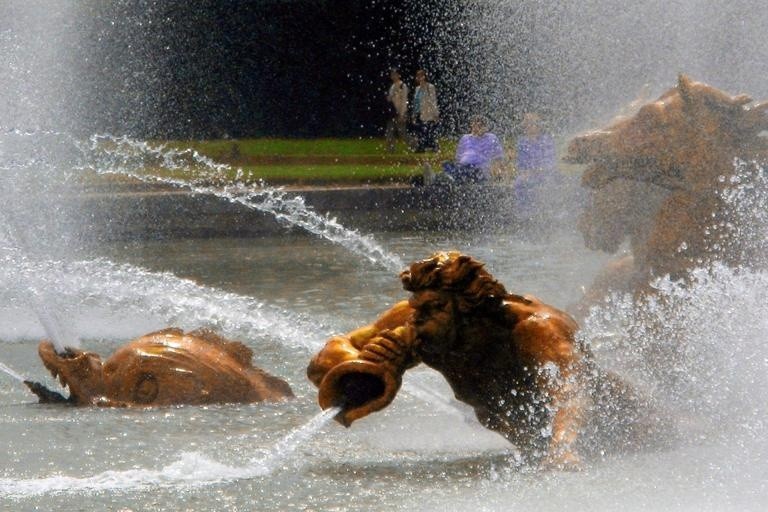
[509,111,557,224]
[384,67,422,154]
[411,69,442,154]
[422,113,506,188]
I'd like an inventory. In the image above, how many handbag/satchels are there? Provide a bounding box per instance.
[384,100,397,118]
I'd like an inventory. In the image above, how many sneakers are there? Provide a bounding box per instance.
[423,162,459,193]
[490,162,518,187]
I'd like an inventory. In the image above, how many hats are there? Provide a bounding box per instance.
[510,113,549,134]
